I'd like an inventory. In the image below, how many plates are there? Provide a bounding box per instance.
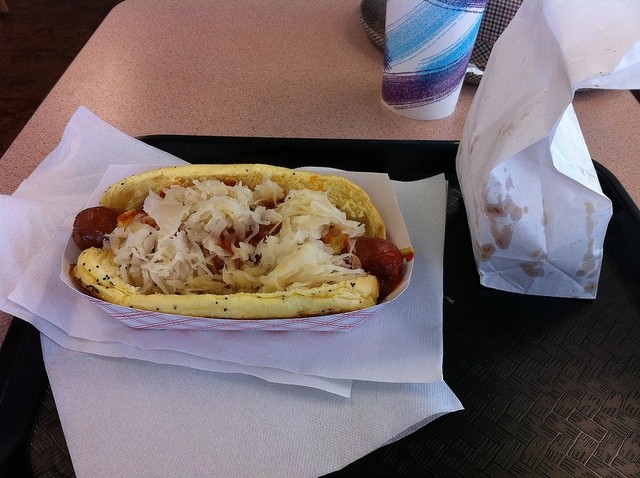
[360,1,596,93]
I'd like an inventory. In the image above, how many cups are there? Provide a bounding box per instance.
[379,1,488,122]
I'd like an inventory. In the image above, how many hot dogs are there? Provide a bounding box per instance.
[69,165,414,321]
